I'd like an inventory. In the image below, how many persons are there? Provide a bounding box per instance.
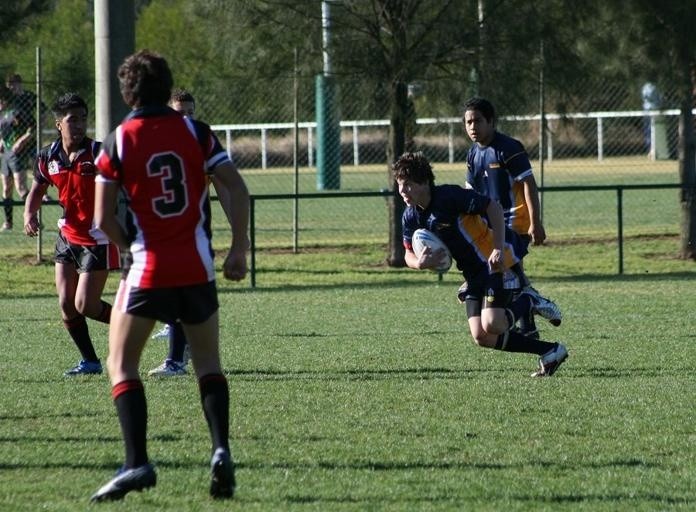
[641,78,658,156]
[463,98,546,339]
[0,74,52,234]
[147,87,196,378]
[23,95,124,380]
[89,49,250,504]
[392,152,569,377]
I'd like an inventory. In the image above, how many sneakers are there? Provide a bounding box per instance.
[91,465,157,504]
[530,342,569,377]
[148,360,187,375]
[211,448,235,499]
[516,324,540,341]
[152,324,170,338]
[0,221,11,231]
[523,287,561,326]
[64,360,102,376]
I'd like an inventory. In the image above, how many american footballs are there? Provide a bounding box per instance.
[412,228,451,270]
